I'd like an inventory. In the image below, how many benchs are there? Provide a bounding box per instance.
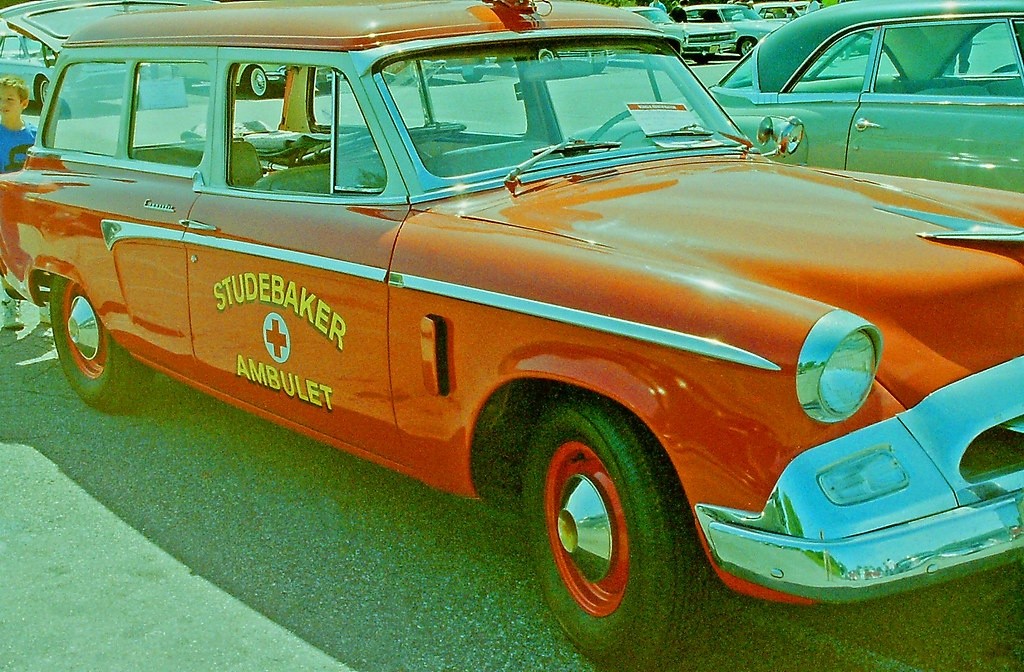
[791,76,908,96]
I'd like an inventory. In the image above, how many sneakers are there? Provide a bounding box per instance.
[2,300,24,329]
[39,307,51,324]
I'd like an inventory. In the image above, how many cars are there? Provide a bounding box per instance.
[175,61,344,102]
[0,29,148,114]
[705,0,1024,195]
[0,0,1024,672]
[681,2,788,57]
[611,5,739,73]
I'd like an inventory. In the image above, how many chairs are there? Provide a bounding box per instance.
[251,164,363,192]
[419,140,478,156]
[914,76,1024,98]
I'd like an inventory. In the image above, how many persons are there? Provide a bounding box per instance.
[0,75,40,333]
